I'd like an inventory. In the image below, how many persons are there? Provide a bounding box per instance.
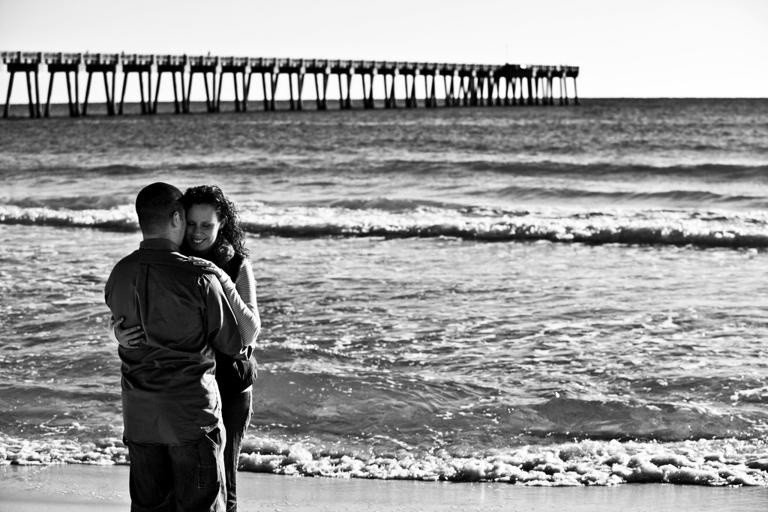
[104,182,255,512]
[108,184,260,512]
